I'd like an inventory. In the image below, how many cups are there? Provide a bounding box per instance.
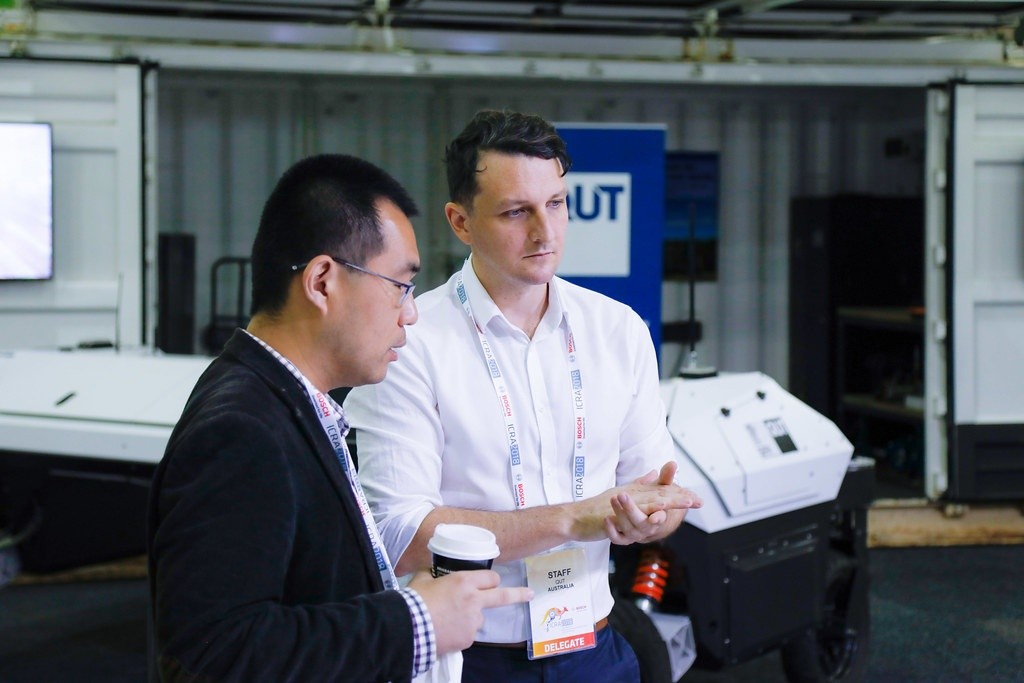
[427,523,500,580]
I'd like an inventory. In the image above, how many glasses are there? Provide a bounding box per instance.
[300,257,416,307]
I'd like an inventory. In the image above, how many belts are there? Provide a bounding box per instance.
[474,616,608,648]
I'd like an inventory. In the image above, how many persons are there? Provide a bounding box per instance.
[343,111,704,683]
[145,153,533,683]
